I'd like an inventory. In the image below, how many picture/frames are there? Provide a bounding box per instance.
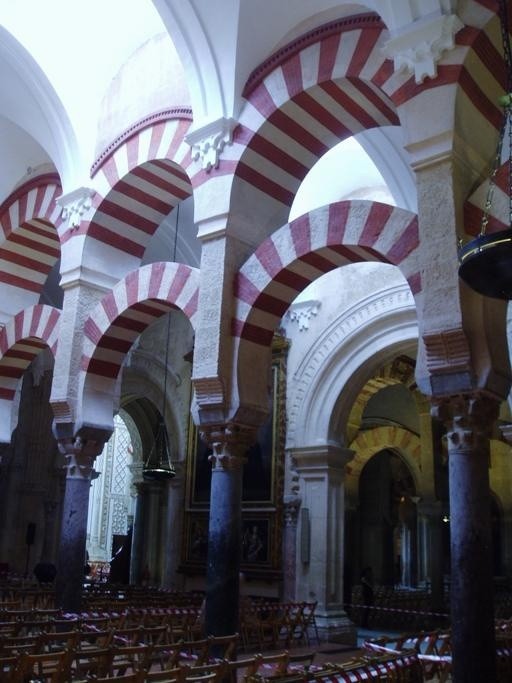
[176,356,287,578]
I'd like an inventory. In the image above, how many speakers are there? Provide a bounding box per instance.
[26,523,37,545]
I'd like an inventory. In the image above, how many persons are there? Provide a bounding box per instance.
[394,554,403,584]
[190,521,264,561]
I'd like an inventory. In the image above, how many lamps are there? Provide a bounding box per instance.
[140,200,183,484]
